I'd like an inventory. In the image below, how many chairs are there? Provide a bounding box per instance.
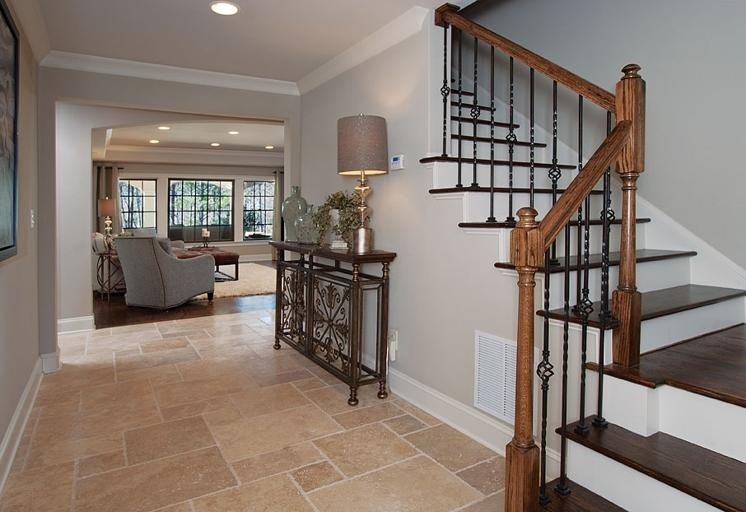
[124,227,184,253]
[115,236,216,311]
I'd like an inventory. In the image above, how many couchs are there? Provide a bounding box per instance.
[92,232,126,293]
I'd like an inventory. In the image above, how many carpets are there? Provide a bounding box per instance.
[191,262,276,303]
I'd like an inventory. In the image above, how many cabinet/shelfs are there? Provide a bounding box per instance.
[269,240,395,406]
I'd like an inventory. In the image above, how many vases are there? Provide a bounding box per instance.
[282,186,306,244]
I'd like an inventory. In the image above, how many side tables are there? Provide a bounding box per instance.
[94,253,126,304]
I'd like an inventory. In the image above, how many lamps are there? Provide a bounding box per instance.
[202,228,210,248]
[337,112,388,253]
